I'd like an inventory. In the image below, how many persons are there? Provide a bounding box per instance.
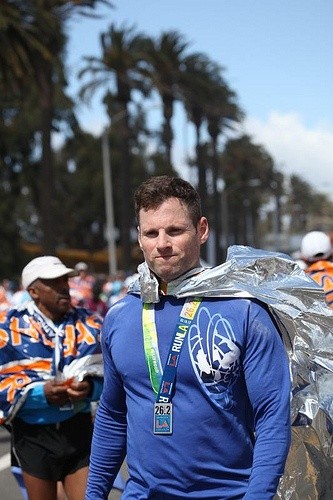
[84,175,291,500]
[0,231,333,500]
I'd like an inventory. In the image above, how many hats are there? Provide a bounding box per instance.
[301,232,333,263]
[11,292,31,311]
[76,262,88,270]
[22,256,78,291]
